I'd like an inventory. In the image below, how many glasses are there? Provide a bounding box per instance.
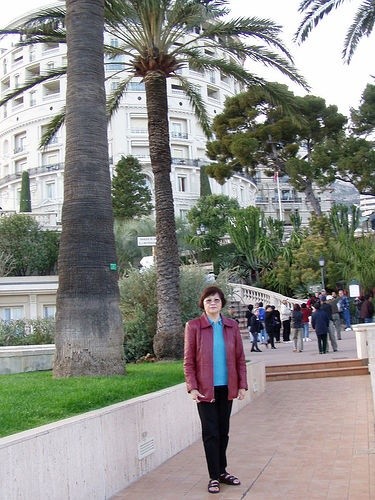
[204,299,220,304]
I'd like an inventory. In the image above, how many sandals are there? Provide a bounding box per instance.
[208,479,220,493]
[218,472,240,485]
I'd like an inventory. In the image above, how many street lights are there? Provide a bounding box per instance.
[264,133,286,224]
[318,254,326,289]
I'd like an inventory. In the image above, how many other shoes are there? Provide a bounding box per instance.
[293,349,297,352]
[265,343,268,348]
[303,338,306,342]
[271,346,277,349]
[306,338,312,341]
[256,348,262,352]
[299,349,303,352]
[333,349,338,352]
[283,341,294,343]
[251,349,258,352]
[275,341,280,343]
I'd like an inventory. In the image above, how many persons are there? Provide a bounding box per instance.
[245,304,257,343]
[318,295,338,353]
[184,287,248,493]
[270,305,282,343]
[353,296,366,324]
[300,303,310,342]
[265,305,278,349]
[310,302,330,353]
[249,308,262,353]
[306,288,353,340]
[252,302,268,344]
[360,294,375,323]
[279,297,293,343]
[290,303,304,352]
